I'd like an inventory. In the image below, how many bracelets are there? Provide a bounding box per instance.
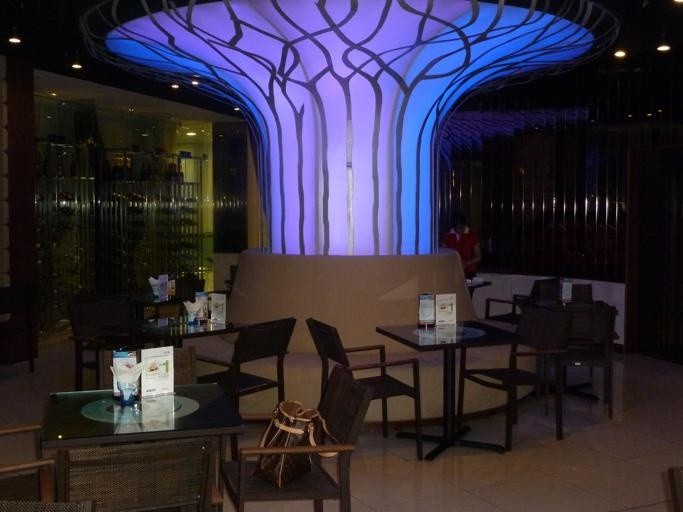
[467,261,472,266]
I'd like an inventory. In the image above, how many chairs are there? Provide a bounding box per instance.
[454,278,619,452]
[94,348,198,388]
[195,318,298,461]
[1,283,44,376]
[219,371,372,512]
[1,423,59,512]
[66,282,244,389]
[305,317,424,465]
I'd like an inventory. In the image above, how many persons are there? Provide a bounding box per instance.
[438,212,482,274]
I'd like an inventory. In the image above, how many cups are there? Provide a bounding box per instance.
[117,379,136,405]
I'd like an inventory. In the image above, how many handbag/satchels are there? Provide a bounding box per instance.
[252,400,342,489]
